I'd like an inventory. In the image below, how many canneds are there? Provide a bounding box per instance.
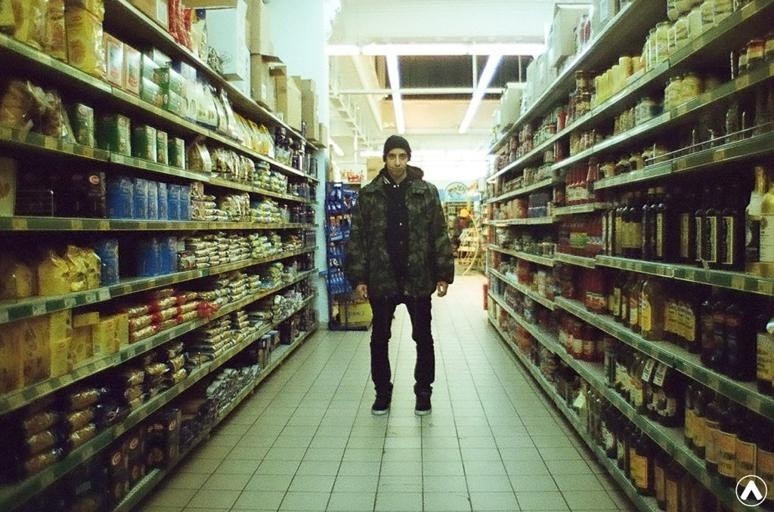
[663,72,701,113]
[644,1,737,70]
[574,70,591,119]
[737,31,773,77]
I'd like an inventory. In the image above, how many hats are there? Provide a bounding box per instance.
[383,135,411,161]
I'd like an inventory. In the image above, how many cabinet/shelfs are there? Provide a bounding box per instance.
[487,0,773,512]
[1,0,320,512]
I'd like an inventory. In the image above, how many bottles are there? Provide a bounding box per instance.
[605,267,773,395]
[526,70,600,219]
[600,1,774,178]
[552,217,606,361]
[602,166,774,278]
[587,334,773,512]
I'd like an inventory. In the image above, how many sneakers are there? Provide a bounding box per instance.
[415,391,432,415]
[372,392,391,415]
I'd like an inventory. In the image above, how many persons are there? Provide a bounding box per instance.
[346,134,456,416]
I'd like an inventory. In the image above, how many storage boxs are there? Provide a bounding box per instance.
[204,0,329,148]
[499,2,625,132]
[128,0,170,31]
